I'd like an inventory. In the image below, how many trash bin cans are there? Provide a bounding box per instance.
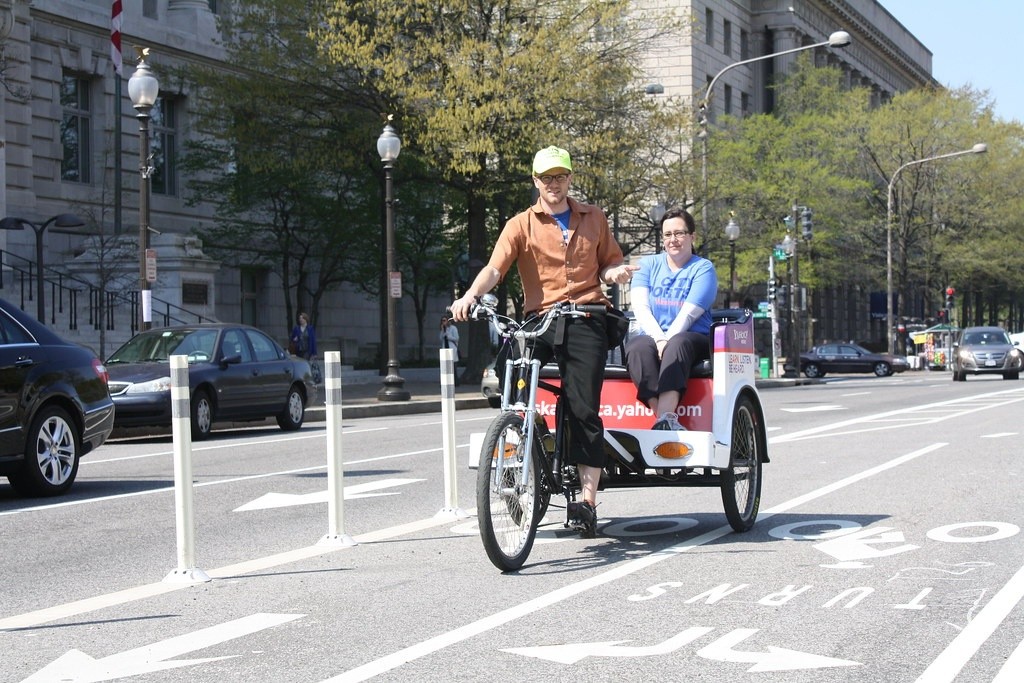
[915,356,923,370]
[907,356,916,371]
[760,357,770,379]
[777,357,791,378]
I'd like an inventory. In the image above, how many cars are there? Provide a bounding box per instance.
[0,297,116,498]
[797,343,910,378]
[952,326,1024,381]
[103,323,316,441]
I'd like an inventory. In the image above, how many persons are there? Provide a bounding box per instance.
[449,145,641,536]
[288,312,319,362]
[989,334,999,342]
[626,208,721,432]
[439,316,460,387]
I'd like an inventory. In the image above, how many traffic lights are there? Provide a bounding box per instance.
[767,280,776,304]
[946,288,955,308]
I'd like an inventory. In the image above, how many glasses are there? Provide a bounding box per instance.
[535,174,570,184]
[663,231,690,239]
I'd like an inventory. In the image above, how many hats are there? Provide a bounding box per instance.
[532,145,572,175]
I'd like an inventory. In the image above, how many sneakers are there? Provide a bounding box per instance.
[515,415,549,463]
[652,412,688,430]
[567,501,598,537]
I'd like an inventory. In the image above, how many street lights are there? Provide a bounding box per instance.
[887,143,988,354]
[609,84,664,363]
[725,212,741,289]
[698,31,852,257]
[376,112,412,402]
[127,45,159,330]
[781,229,798,377]
[0,212,86,325]
[649,205,665,255]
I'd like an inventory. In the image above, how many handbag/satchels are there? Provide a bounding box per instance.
[311,359,322,383]
[457,347,461,359]
[607,308,630,350]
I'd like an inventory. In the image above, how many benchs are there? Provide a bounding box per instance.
[525,308,748,378]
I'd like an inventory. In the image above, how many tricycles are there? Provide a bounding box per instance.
[443,294,770,572]
